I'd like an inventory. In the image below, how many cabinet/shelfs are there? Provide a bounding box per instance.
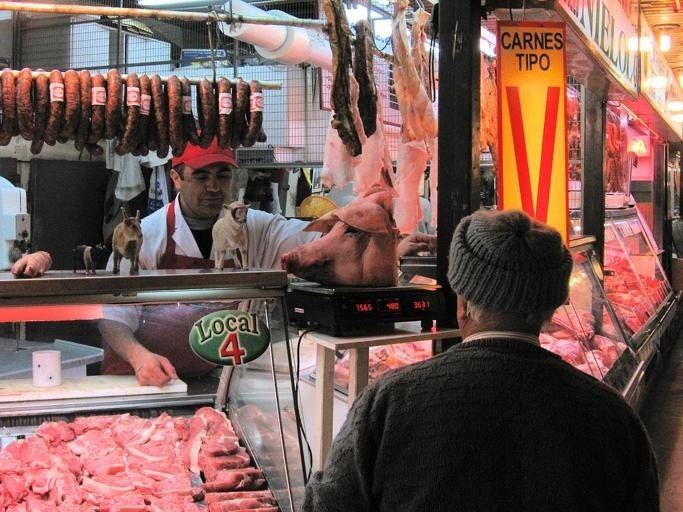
[295,229,645,474]
[557,197,679,400]
[0,262,316,512]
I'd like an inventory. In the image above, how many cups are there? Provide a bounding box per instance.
[31,350,63,388]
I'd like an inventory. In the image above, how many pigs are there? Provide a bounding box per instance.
[280,190,399,287]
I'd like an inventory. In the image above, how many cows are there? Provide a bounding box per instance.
[69,243,110,275]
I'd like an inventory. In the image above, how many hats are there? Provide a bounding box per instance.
[447,211,574,314]
[172,137,239,170]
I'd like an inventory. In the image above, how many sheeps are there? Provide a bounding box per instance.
[211,201,250,270]
[112,207,142,275]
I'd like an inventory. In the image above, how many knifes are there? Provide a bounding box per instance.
[189,432,206,501]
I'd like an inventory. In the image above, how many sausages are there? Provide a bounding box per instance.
[0,67,266,156]
[606,121,626,192]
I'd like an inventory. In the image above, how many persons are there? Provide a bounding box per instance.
[298,205,665,510]
[92,126,437,387]
